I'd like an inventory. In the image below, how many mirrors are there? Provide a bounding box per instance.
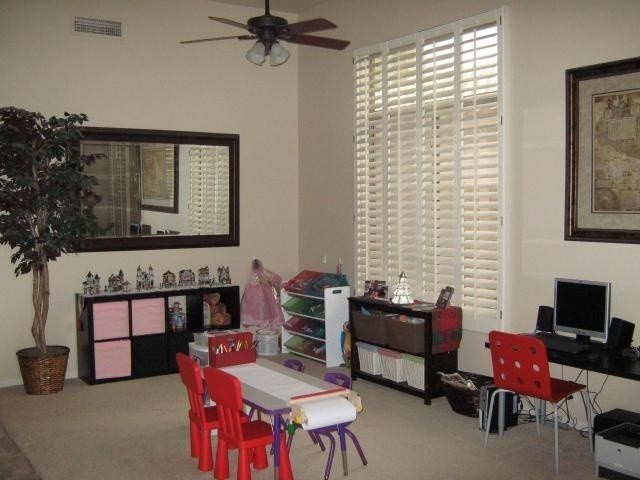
[64,125,242,253]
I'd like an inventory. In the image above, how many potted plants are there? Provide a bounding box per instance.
[0,105,109,395]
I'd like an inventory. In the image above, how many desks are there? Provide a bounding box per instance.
[485,331,639,437]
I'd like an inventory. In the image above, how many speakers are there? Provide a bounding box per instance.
[609,317,634,347]
[535,306,555,337]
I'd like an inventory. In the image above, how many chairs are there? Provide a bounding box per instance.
[176,333,369,480]
[482,330,593,473]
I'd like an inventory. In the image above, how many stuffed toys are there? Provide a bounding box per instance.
[203,291,232,326]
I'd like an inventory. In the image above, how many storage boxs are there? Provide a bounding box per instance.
[347,295,459,404]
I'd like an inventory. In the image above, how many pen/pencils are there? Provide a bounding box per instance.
[215,340,259,355]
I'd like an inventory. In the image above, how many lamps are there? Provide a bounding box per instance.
[244,40,291,66]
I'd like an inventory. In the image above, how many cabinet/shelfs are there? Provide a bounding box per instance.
[75,283,240,387]
[279,270,351,369]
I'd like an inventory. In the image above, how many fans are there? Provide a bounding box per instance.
[178,0,357,51]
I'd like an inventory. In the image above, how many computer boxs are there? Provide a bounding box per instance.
[478,383,519,432]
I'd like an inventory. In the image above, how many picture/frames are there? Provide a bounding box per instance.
[564,55,640,244]
[137,144,179,214]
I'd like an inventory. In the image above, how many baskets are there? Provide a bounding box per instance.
[438,370,494,417]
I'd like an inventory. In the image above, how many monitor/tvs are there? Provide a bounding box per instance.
[553,278,611,343]
